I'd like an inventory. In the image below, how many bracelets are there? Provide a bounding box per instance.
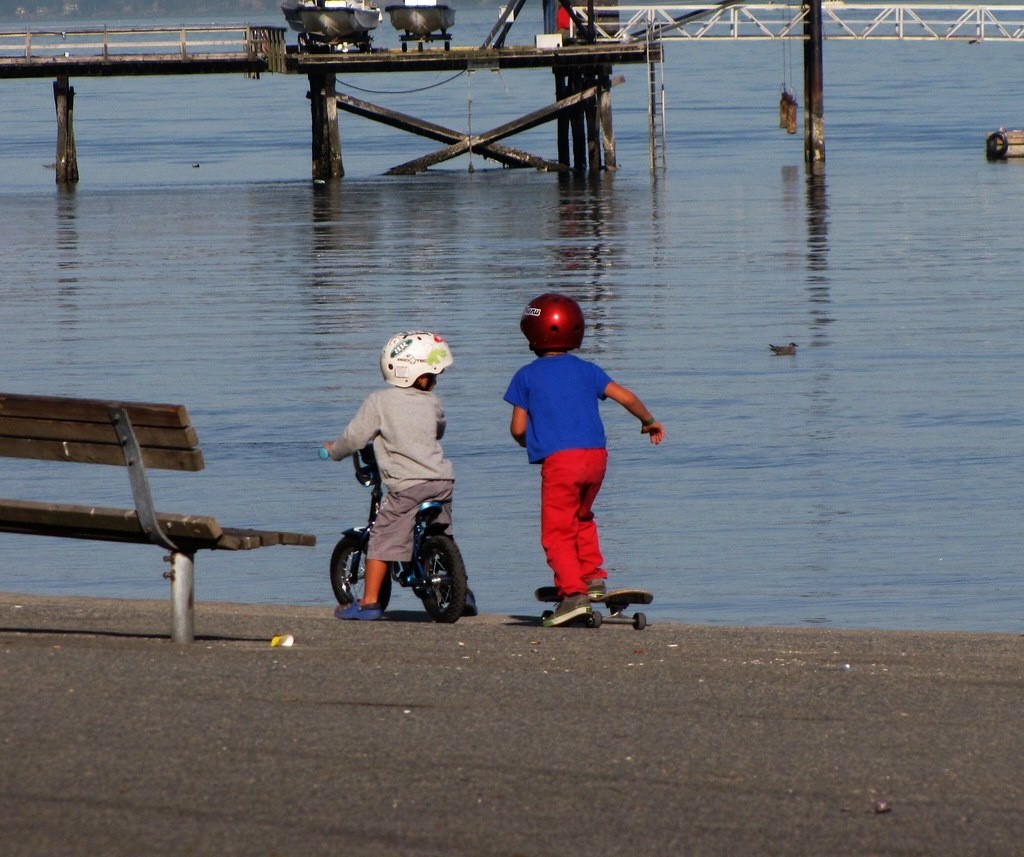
[642,417,656,425]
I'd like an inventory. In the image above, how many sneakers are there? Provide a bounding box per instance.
[542,593,592,627]
[586,579,606,598]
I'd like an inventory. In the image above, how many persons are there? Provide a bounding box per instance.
[503,291,667,628]
[322,328,479,621]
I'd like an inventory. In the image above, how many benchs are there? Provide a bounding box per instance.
[0,392,318,646]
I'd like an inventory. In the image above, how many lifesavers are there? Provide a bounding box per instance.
[985,131,1010,162]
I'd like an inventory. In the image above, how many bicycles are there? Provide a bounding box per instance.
[317,443,469,627]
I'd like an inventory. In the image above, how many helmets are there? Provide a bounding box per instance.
[520,294,584,350]
[380,330,454,388]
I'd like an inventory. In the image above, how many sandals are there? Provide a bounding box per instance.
[333,598,382,620]
[462,588,477,617]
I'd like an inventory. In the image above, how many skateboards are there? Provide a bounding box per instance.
[533,582,655,631]
